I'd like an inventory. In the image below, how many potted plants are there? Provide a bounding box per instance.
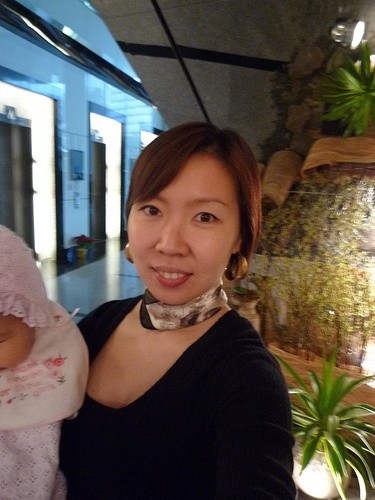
[276,345,375,499]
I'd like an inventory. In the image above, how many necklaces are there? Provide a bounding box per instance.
[139,277,227,332]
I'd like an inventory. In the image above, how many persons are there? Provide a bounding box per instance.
[58,121,298,500]
[1,224,89,500]
